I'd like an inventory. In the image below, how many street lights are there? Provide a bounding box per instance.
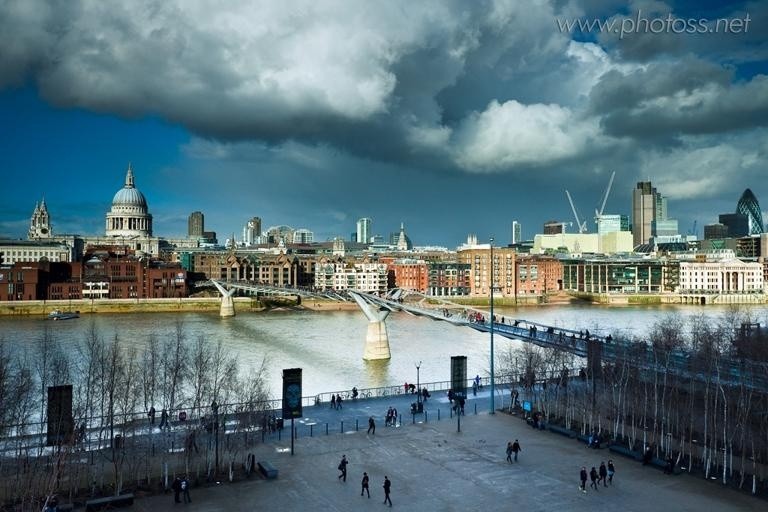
[210,401,219,472]
[414,360,422,410]
[488,237,495,414]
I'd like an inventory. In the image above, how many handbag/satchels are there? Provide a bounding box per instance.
[607,469,613,475]
[337,464,344,471]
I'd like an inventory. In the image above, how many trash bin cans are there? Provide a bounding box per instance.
[276,418,283,429]
[411,403,416,415]
[115,434,121,447]
[418,402,423,412]
[527,417,533,425]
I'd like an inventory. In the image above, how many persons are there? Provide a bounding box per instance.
[502,317,504,323]
[606,334,612,343]
[472,375,481,396]
[558,331,569,343]
[493,315,496,321]
[158,409,169,428]
[74,424,88,442]
[506,439,522,464]
[515,320,518,326]
[663,458,675,475]
[532,412,543,430]
[529,325,537,339]
[338,455,348,482]
[361,471,371,498]
[547,327,553,334]
[404,383,415,392]
[570,334,576,345]
[423,387,429,401]
[331,394,342,411]
[578,329,590,341]
[443,308,481,324]
[268,414,276,434]
[639,446,652,466]
[448,391,465,413]
[578,459,615,494]
[579,369,586,381]
[640,341,649,351]
[171,474,193,504]
[386,406,397,424]
[39,497,58,512]
[352,387,358,400]
[383,475,392,507]
[147,406,155,425]
[368,416,375,435]
[588,428,601,449]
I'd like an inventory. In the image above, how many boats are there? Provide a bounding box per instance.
[48,309,80,322]
